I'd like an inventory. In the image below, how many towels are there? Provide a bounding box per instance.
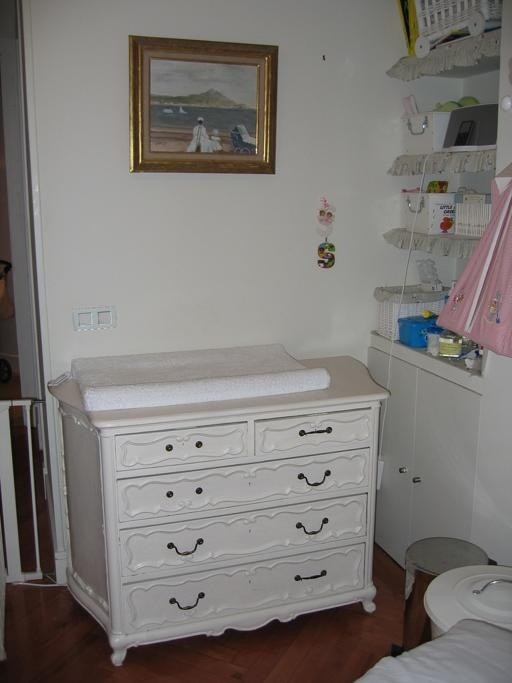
[73,342,333,412]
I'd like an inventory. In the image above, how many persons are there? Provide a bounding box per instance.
[210,129,224,149]
[186,116,211,153]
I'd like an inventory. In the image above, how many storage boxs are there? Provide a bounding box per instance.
[402,109,452,154]
[401,191,456,235]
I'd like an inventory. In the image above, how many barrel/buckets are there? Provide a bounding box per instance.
[423,565,512,642]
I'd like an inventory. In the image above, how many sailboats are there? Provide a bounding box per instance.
[176,104,188,114]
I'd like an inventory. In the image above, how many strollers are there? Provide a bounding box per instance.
[229,124,256,153]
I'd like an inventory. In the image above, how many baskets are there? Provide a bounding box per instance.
[415,0,501,44]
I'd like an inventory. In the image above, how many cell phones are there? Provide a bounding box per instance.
[454,121,473,144]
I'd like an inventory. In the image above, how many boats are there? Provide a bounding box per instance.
[163,108,174,113]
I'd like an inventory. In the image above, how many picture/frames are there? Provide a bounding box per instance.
[127,33,278,175]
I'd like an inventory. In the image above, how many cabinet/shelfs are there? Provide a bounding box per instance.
[47,353,391,668]
[384,25,499,256]
[365,345,512,573]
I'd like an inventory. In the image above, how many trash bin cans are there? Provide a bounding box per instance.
[399,535,492,653]
